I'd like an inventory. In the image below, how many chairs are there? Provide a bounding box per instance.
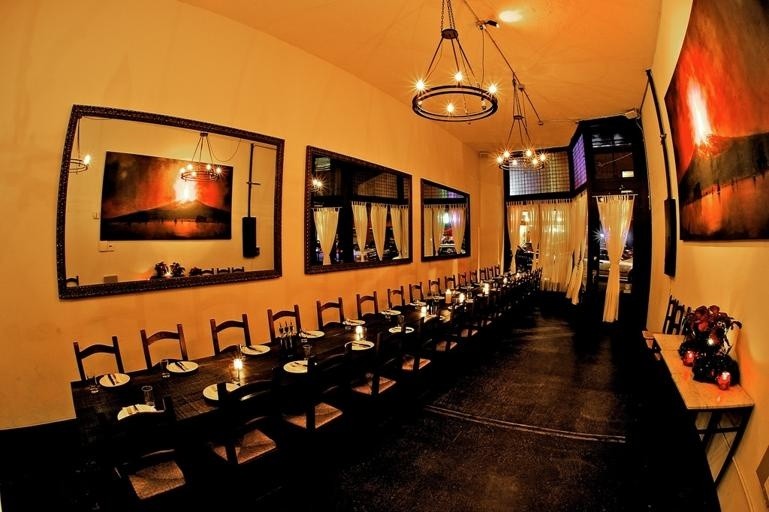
[66,276,78,287]
[642,295,694,361]
[200,267,244,276]
[70,264,542,512]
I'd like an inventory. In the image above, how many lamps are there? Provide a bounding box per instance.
[68,115,88,174]
[412,0,549,171]
[181,133,220,182]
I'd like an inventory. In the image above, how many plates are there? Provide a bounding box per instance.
[282,360,317,374]
[167,362,199,373]
[241,346,271,355]
[100,373,131,387]
[294,274,530,359]
[118,404,157,420]
[203,383,240,400]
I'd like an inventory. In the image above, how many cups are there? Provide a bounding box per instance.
[86,373,98,394]
[160,359,171,378]
[142,385,155,409]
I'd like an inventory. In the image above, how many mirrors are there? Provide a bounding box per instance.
[56,105,285,298]
[304,146,412,274]
[421,178,471,262]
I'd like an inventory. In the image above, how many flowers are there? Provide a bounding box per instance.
[678,305,741,390]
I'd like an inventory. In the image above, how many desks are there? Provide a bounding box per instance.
[646,334,756,507]
